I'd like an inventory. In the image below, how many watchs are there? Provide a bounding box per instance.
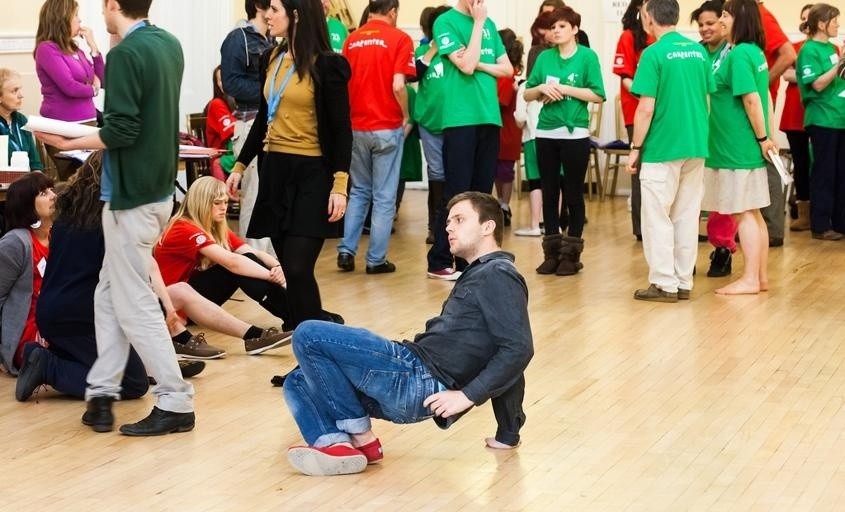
[629,142,642,151]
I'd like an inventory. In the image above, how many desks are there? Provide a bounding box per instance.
[54,145,222,216]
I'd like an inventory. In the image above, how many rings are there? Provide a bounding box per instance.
[341,210,344,217]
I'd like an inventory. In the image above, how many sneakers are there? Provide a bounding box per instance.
[171,332,227,360]
[812,230,844,242]
[426,267,463,282]
[337,252,355,271]
[15,348,49,402]
[181,360,205,378]
[513,229,541,237]
[365,259,396,274]
[502,208,512,227]
[19,342,46,374]
[243,328,296,356]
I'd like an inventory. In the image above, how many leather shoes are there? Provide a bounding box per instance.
[679,288,690,300]
[767,237,784,247]
[634,286,678,304]
[82,397,116,433]
[118,408,196,438]
[706,247,733,277]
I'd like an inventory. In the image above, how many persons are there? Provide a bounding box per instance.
[204,67,236,183]
[152,253,292,362]
[0,172,205,381]
[425,1,514,282]
[413,6,455,245]
[225,0,353,331]
[0,68,59,180]
[81,0,196,437]
[416,6,434,46]
[152,176,345,332]
[756,0,798,248]
[220,0,278,238]
[794,0,845,241]
[495,25,524,227]
[779,5,842,231]
[626,0,718,303]
[322,0,349,55]
[612,1,658,242]
[33,0,102,129]
[524,5,606,275]
[356,5,424,235]
[700,1,778,295]
[690,1,731,70]
[283,192,534,476]
[336,0,416,275]
[15,147,150,401]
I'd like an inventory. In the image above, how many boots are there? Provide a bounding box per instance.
[534,234,563,273]
[556,236,585,276]
[789,199,811,232]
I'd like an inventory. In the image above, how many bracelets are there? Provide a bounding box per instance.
[755,136,768,143]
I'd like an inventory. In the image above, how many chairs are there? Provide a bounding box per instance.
[186,113,242,220]
[588,102,603,202]
[601,93,633,204]
